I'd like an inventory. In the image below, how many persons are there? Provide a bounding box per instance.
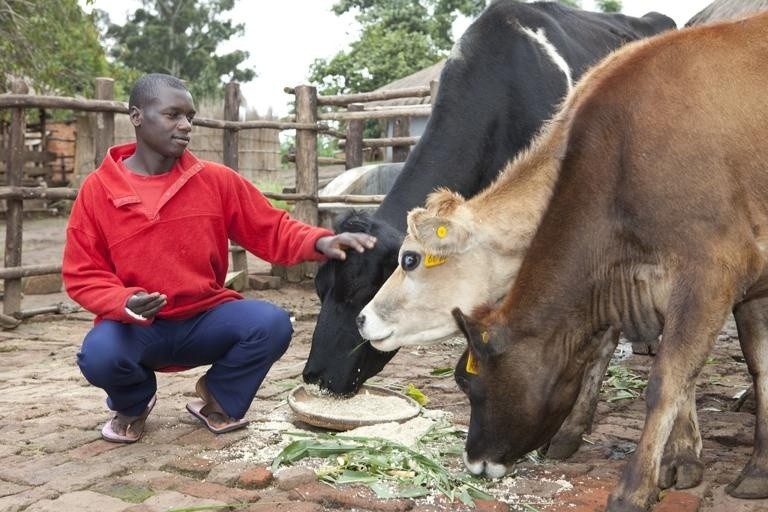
[62,71,377,444]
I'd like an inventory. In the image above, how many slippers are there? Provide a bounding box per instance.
[185,397,249,435]
[100,394,160,444]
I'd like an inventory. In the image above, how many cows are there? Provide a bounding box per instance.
[302,0,678,396]
[353,2,768,490]
[451,6,767,512]
